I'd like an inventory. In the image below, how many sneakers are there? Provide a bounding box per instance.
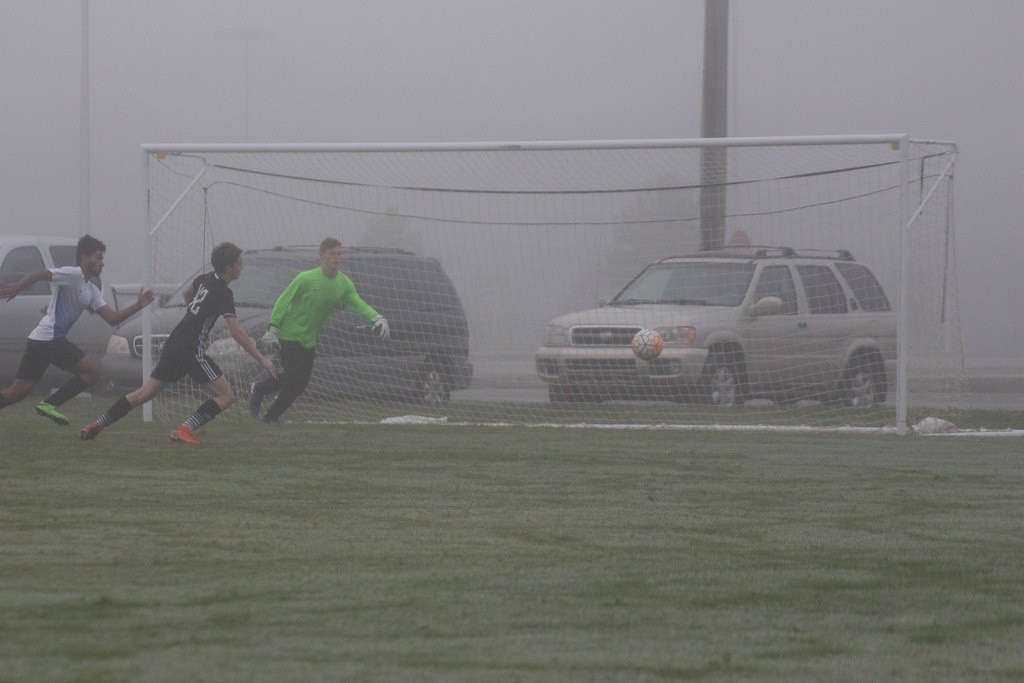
[80,422,99,441]
[170,428,202,445]
[37,400,70,426]
[250,381,263,417]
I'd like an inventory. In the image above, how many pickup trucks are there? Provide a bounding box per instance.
[1,236,192,395]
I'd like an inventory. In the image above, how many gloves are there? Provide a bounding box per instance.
[262,324,283,355]
[372,315,390,341]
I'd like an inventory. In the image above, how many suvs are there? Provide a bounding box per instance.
[107,241,475,406]
[535,243,897,410]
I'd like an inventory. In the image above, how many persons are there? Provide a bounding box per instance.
[2,234,156,425]
[78,241,277,446]
[249,237,389,424]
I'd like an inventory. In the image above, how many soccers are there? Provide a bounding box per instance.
[632,329,664,361]
